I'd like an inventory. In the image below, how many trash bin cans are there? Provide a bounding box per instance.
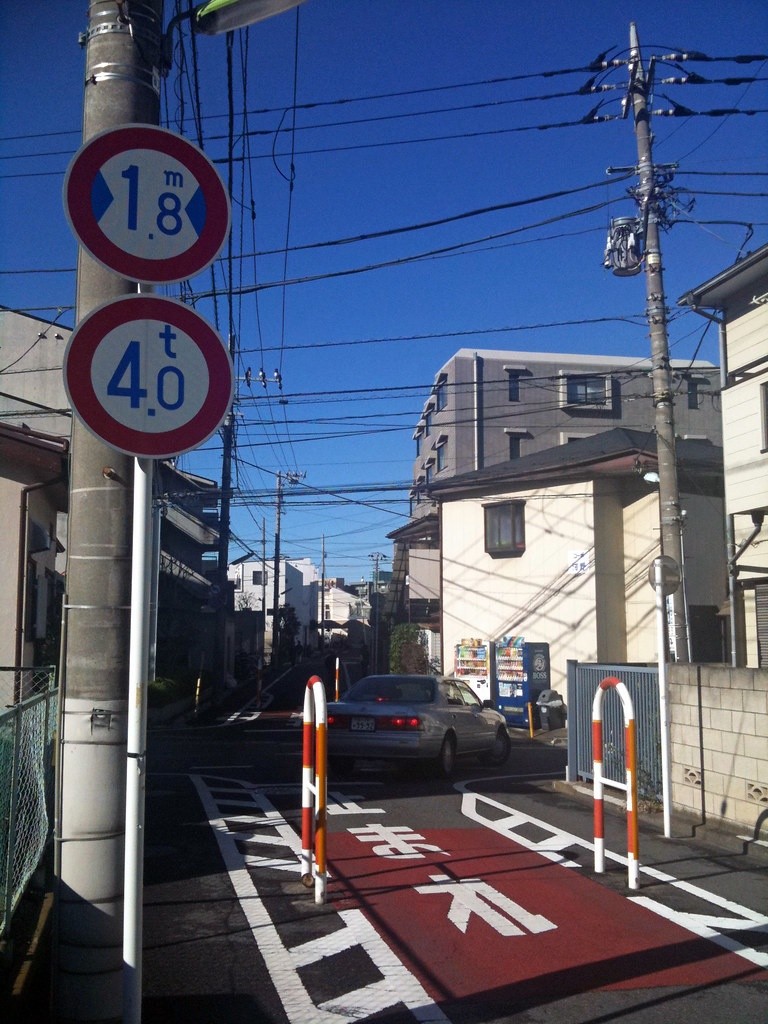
[536,690,566,732]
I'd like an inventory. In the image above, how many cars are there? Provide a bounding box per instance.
[316,674,511,778]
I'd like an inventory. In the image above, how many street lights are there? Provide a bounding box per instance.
[118,2,306,1024]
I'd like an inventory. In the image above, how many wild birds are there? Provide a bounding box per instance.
[258,368,266,389]
[245,366,252,388]
[273,368,282,390]
